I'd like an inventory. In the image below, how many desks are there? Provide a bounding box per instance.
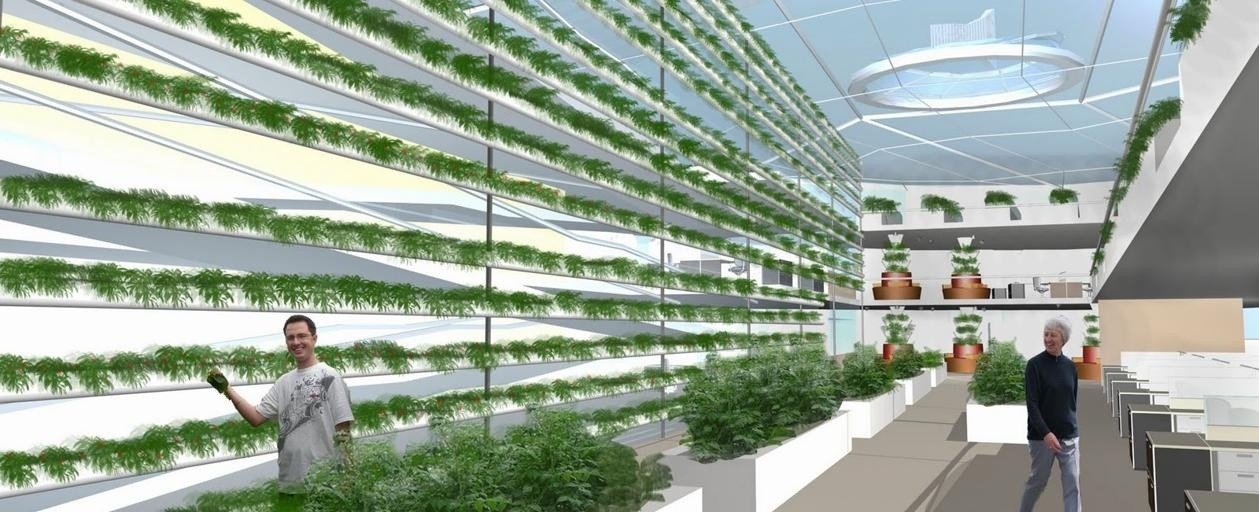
[1048,282,1083,298]
[1101,365,1259,512]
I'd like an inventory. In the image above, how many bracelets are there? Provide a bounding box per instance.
[234,398,243,407]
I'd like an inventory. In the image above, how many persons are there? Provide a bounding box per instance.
[205,312,357,512]
[1014,313,1084,511]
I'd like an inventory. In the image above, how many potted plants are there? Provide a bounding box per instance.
[1161,0,1214,125]
[842,345,904,439]
[942,311,984,360]
[901,193,966,224]
[860,196,902,225]
[880,313,914,361]
[659,356,852,508]
[1081,314,1099,363]
[1017,188,1079,220]
[920,348,948,387]
[962,190,1019,221]
[950,241,982,287]
[298,412,702,510]
[879,241,914,287]
[894,342,928,405]
[965,335,1030,445]
[1103,92,1184,227]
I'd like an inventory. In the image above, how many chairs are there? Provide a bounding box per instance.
[1033,276,1051,293]
[1081,283,1093,298]
